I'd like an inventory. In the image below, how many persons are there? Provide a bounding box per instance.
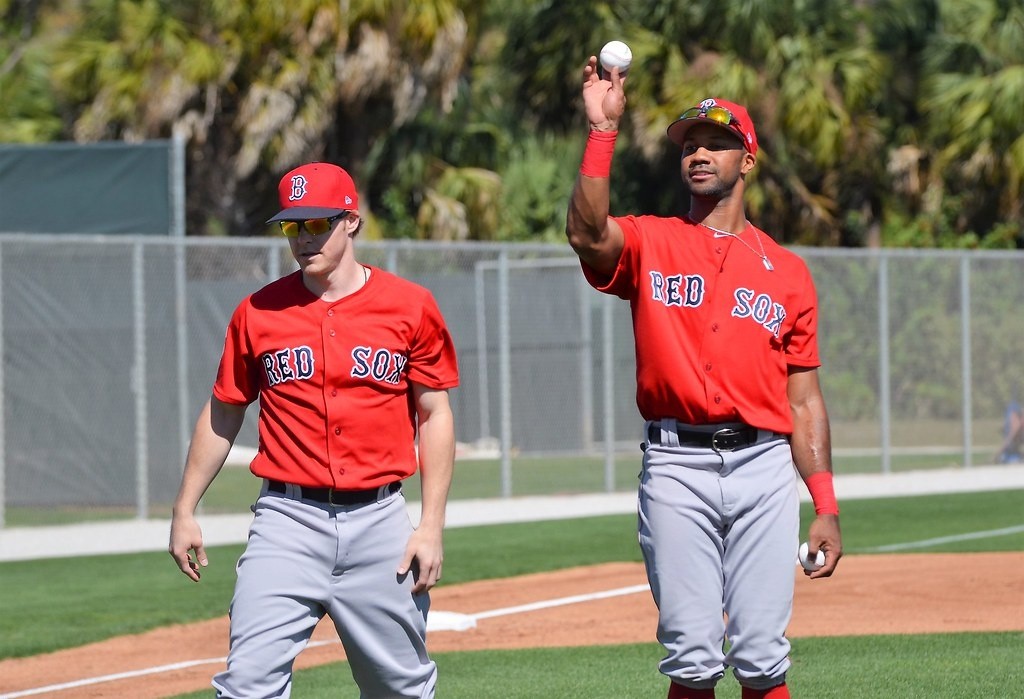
[564,55,841,699]
[167,161,461,699]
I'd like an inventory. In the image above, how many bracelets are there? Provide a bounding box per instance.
[804,471,840,517]
[577,129,616,185]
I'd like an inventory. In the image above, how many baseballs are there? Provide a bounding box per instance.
[600,40,633,74]
[798,541,826,572]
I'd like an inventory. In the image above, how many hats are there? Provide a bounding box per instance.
[265,160,359,225]
[667,98,758,158]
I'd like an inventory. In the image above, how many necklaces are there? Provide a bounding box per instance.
[361,266,369,284]
[685,209,774,272]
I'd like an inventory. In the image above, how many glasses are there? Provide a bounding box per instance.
[678,106,740,124]
[279,210,349,238]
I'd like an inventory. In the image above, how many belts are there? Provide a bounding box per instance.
[267,479,402,506]
[648,425,780,452]
